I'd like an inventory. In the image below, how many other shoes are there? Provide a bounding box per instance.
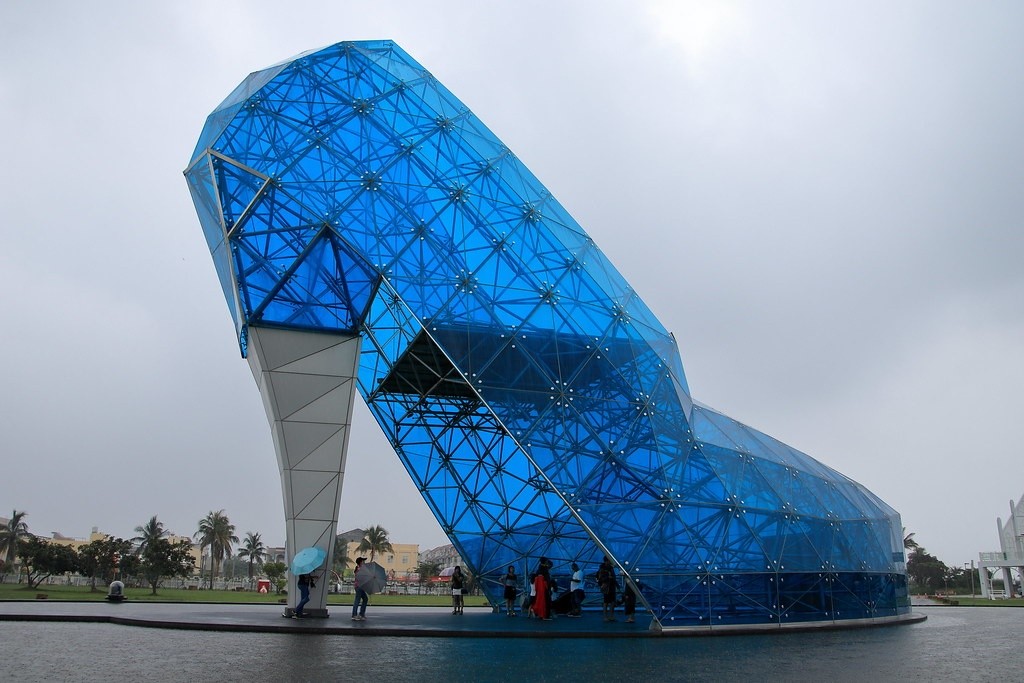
[358,615,367,620]
[351,616,360,620]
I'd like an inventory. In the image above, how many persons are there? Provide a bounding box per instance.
[351,557,369,621]
[521,555,563,622]
[596,555,619,624]
[499,565,520,618]
[567,563,586,619]
[451,566,465,616]
[291,573,320,619]
[618,576,637,625]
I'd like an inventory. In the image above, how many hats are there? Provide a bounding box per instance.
[356,557,367,562]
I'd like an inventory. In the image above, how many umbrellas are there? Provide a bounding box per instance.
[357,561,387,595]
[290,546,327,576]
[438,566,465,576]
